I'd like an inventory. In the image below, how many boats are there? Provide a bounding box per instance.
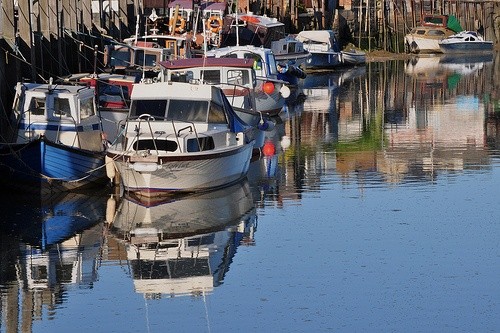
[294,30,340,68]
[53,0,317,145]
[0,77,117,196]
[104,187,258,300]
[0,170,122,293]
[437,30,494,54]
[104,80,256,197]
[403,14,464,54]
[338,49,367,66]
